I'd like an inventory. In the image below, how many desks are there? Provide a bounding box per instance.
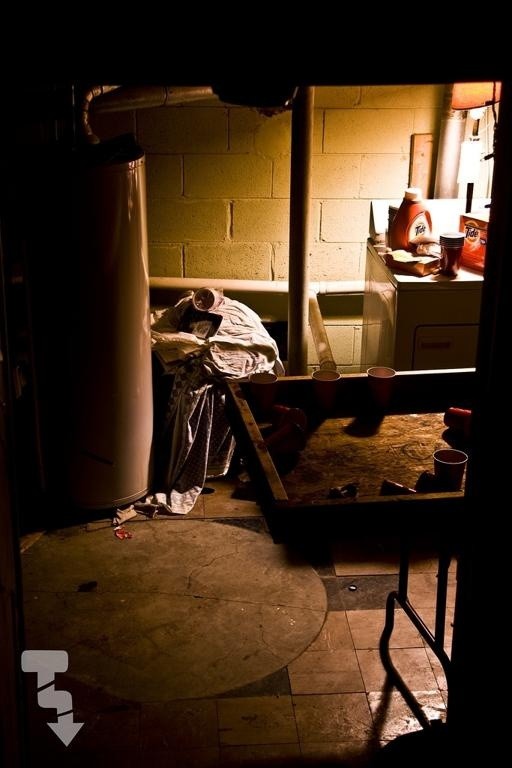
[223,367,475,721]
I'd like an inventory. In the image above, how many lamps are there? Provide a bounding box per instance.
[452,82,501,120]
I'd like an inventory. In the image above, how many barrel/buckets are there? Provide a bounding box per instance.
[389,186,433,250]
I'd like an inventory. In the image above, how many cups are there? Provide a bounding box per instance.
[439,234,465,276]
[441,408,472,432]
[434,448,466,489]
[248,372,278,405]
[310,369,342,411]
[365,367,396,415]
